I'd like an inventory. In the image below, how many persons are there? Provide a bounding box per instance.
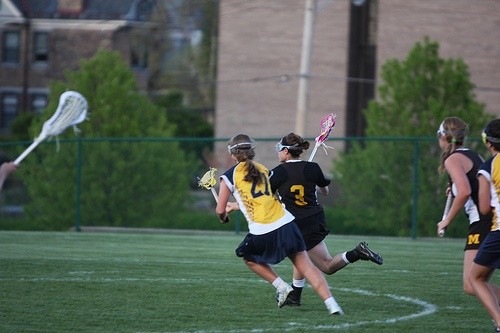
[268,133,384,307]
[437,116,500,306]
[215,134,346,319]
[466,119,500,333]
[0,161,19,191]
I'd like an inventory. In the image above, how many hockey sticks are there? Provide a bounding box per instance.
[196,165,230,223]
[13,91,89,166]
[307,113,335,162]
[438,191,452,238]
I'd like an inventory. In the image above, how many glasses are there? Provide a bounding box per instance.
[227,146,233,155]
[275,143,284,151]
[481,130,487,143]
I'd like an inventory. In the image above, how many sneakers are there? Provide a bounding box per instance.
[330,305,344,315]
[276,294,299,306]
[275,284,294,308]
[356,241,383,265]
